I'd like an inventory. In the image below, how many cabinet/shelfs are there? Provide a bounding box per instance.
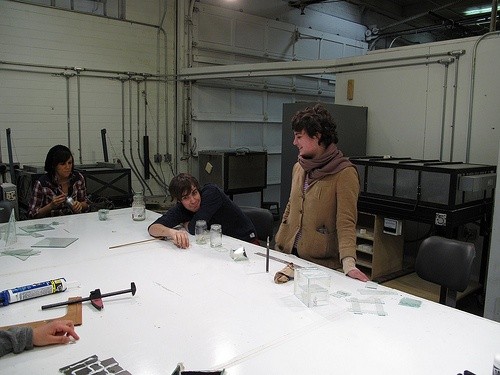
[357,211,406,280]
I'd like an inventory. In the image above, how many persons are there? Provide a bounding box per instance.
[275,104,369,282]
[0,320,80,358]
[28,145,89,219]
[148,173,260,249]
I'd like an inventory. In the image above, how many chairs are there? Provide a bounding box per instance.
[414,236,476,307]
[240,206,274,246]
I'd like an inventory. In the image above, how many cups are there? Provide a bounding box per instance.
[195,220,207,244]
[210,224,223,247]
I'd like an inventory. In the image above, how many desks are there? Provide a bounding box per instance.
[0,208,500,375]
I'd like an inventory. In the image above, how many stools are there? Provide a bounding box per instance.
[263,201,280,221]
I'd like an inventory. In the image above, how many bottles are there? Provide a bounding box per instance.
[66,196,75,207]
[0,277,67,307]
[132,192,145,221]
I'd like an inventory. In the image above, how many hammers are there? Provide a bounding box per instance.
[41,281,137,311]
